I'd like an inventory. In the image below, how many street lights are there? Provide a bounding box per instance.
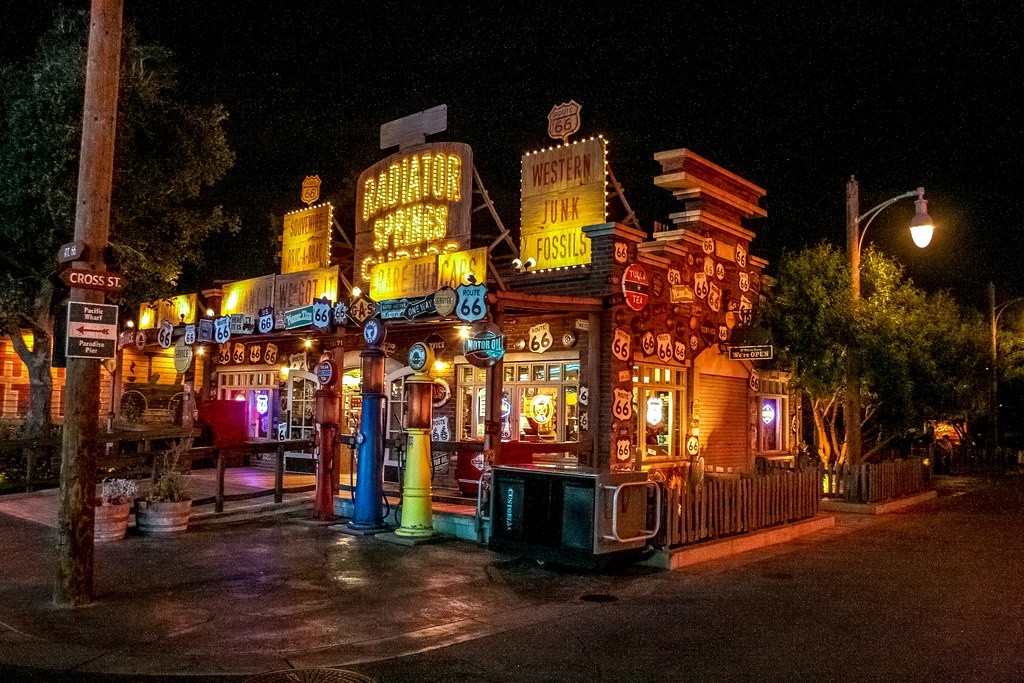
[844,173,936,504]
